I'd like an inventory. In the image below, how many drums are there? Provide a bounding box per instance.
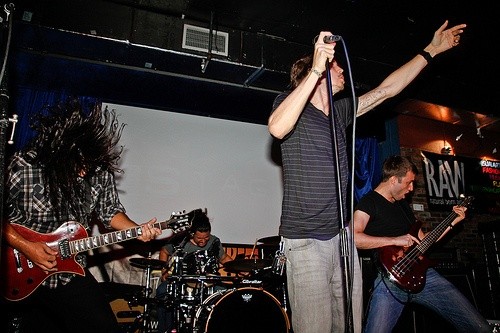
[193,286,291,333]
[168,274,239,330]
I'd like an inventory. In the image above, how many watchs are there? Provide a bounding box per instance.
[419,49,433,64]
[450,223,454,229]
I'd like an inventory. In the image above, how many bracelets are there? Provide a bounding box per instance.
[311,69,322,77]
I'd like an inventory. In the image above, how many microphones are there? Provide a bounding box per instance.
[311,34,341,45]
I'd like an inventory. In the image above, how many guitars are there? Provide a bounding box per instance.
[374,195,475,294]
[5,210,190,301]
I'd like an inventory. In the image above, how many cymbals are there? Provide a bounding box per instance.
[258,236,281,244]
[128,258,168,269]
[223,259,272,273]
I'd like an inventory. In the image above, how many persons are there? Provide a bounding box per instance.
[3,97,162,333]
[347,154,493,333]
[267,18,466,333]
[160,212,234,296]
